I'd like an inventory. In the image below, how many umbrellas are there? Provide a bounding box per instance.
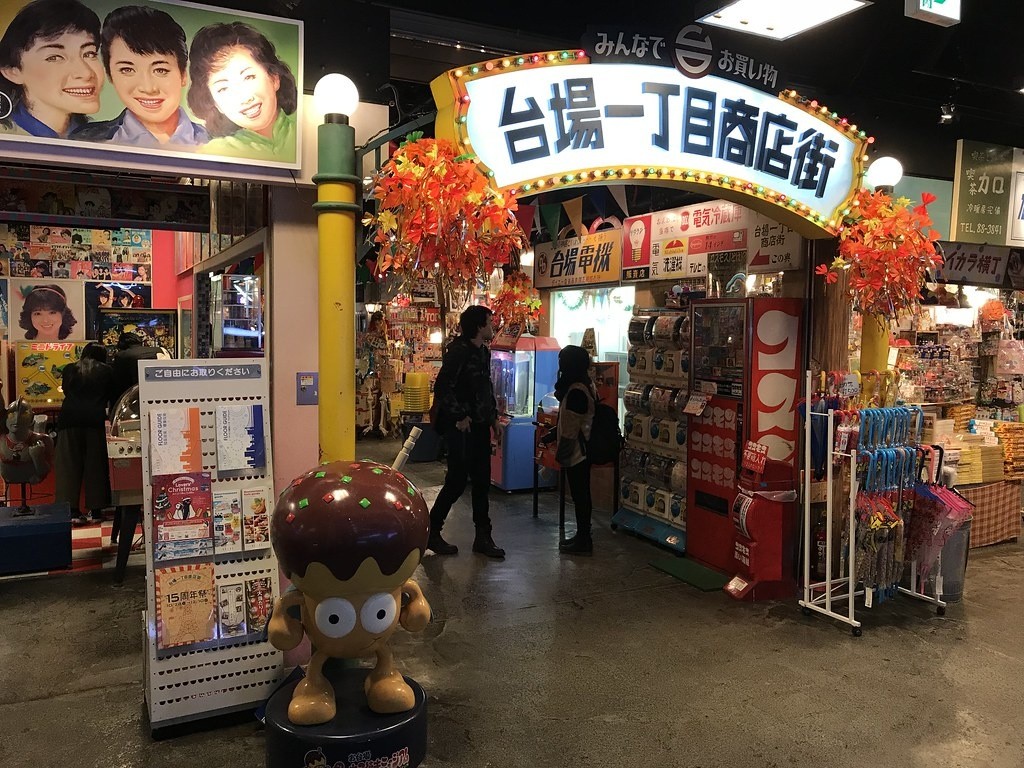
[795,370,977,604]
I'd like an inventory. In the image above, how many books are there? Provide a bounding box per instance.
[910,404,1024,483]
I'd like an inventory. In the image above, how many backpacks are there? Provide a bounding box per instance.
[557,381,627,466]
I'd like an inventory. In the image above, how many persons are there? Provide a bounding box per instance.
[18,282,136,342]
[53,332,173,524]
[426,305,506,557]
[267,461,431,725]
[0,394,53,485]
[0,227,152,282]
[541,345,597,557]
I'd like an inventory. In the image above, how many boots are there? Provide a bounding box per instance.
[471,524,507,558]
[426,520,459,555]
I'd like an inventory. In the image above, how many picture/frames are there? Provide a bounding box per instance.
[98,307,178,359]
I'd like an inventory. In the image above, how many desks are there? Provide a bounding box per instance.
[953,480,1022,548]
[110,491,144,587]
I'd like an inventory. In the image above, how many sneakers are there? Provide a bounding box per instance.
[91,509,103,525]
[558,523,594,557]
[108,567,127,592]
[69,510,88,526]
[109,539,120,548]
